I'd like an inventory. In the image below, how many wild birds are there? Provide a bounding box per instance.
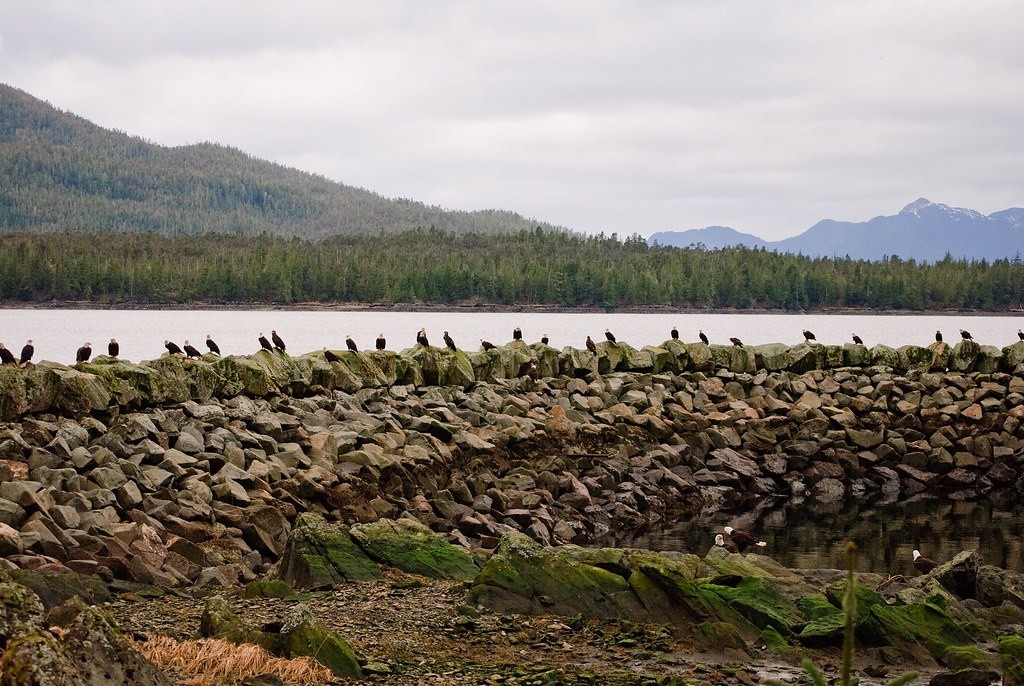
[376,333,387,351]
[851,332,864,345]
[730,337,744,348]
[935,330,943,342]
[604,329,616,344]
[206,334,221,356]
[698,329,709,346]
[802,329,816,342]
[18,339,34,366]
[258,330,286,353]
[959,328,974,342]
[416,328,596,353]
[1017,328,1024,342]
[76,342,93,361]
[323,334,358,362]
[0,343,17,366]
[183,340,203,358]
[108,339,120,358]
[671,326,680,340]
[164,340,185,356]
